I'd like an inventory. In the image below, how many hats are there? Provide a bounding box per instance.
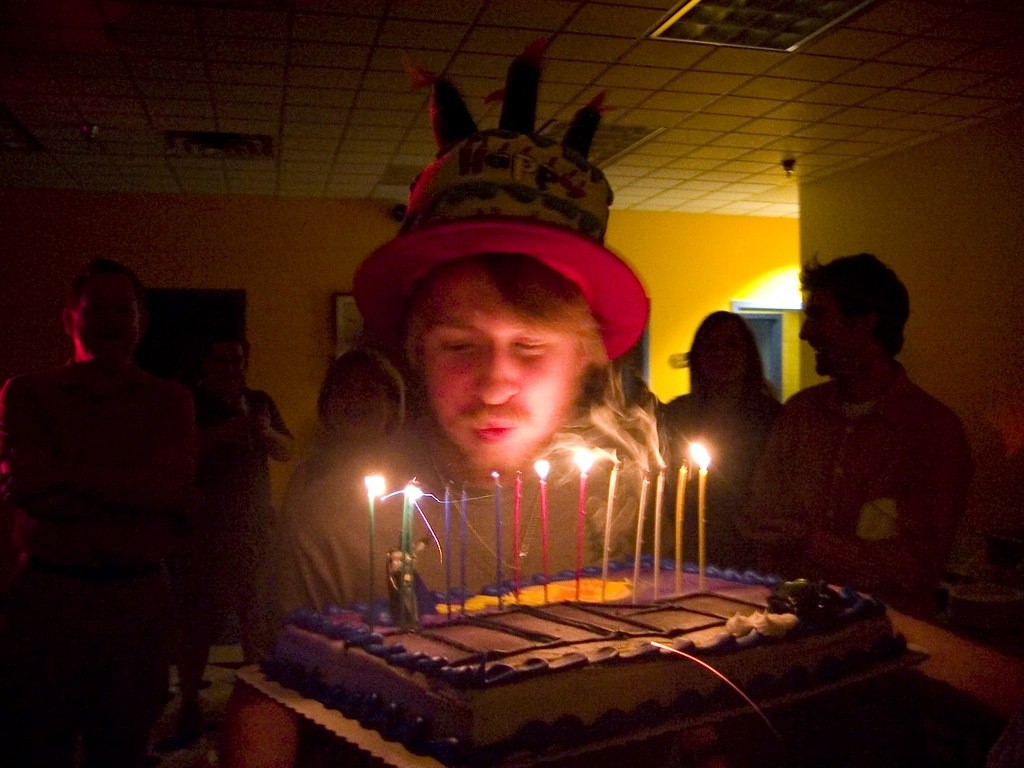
[351,35,650,359]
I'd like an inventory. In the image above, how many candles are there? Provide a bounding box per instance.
[445,484,451,614]
[602,461,619,599]
[368,493,375,597]
[538,475,549,593]
[698,464,709,589]
[575,470,587,600]
[675,460,690,596]
[653,468,666,599]
[631,470,650,600]
[513,471,523,602]
[460,482,469,614]
[495,473,505,611]
[400,496,416,627]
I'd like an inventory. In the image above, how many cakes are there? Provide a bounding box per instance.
[273,554,906,768]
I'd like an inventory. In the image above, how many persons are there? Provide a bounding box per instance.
[241,50,668,663]
[730,253,972,593]
[276,346,405,514]
[660,309,786,573]
[195,333,295,608]
[0,260,198,768]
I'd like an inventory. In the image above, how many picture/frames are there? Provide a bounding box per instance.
[334,293,365,352]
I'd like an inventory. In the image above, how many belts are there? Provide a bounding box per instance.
[28,563,162,588]
